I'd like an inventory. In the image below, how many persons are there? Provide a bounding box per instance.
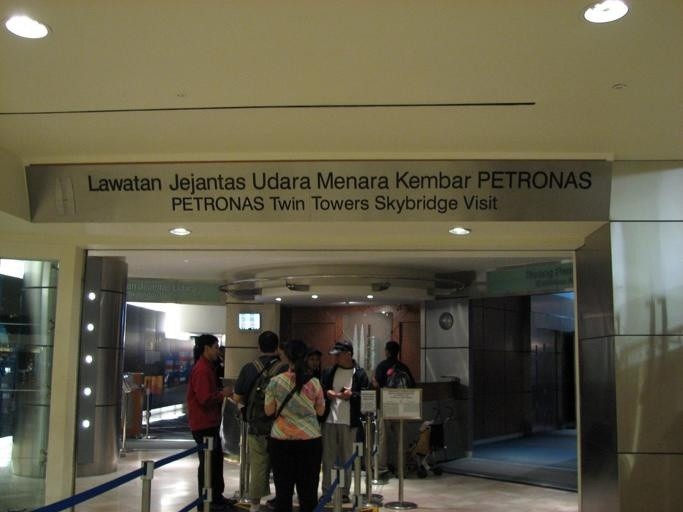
[375,340,415,387]
[317,339,370,502]
[233,331,325,512]
[187,332,238,512]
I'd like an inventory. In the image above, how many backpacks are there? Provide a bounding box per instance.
[386,365,412,387]
[245,361,285,434]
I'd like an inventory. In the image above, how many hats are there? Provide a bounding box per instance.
[328,341,353,355]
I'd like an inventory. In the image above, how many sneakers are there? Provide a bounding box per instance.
[319,494,349,503]
[197,497,277,512]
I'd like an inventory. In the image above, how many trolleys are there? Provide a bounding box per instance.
[396,376,467,478]
